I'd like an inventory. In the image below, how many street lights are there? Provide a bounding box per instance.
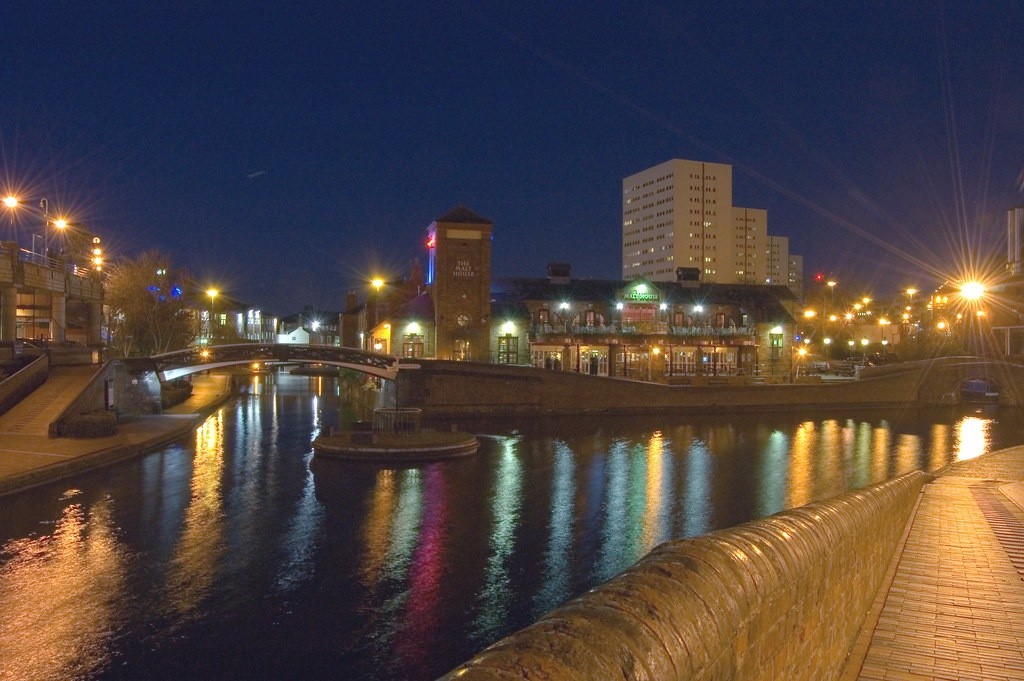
[879,318,887,340]
[849,341,853,372]
[882,341,888,367]
[828,282,836,306]
[823,338,830,375]
[863,298,869,311]
[207,288,218,314]
[32,233,43,254]
[371,278,383,327]
[861,339,869,366]
[907,288,915,305]
[40,197,50,257]
